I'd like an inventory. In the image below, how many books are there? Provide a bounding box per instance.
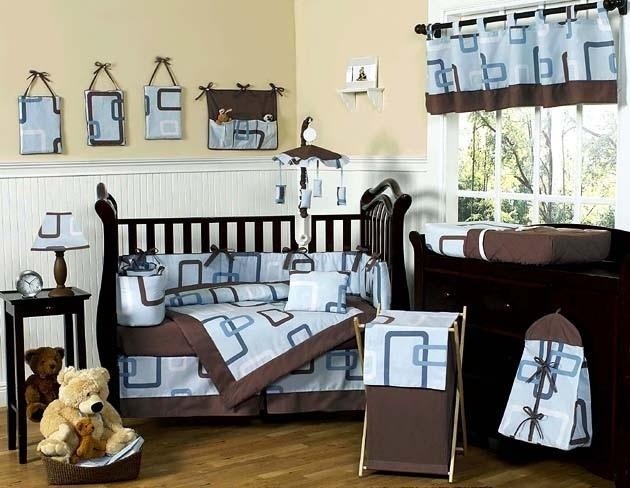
[105,436,144,466]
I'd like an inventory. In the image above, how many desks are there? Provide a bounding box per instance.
[0,287,92,464]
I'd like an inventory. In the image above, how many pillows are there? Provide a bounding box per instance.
[284,269,350,314]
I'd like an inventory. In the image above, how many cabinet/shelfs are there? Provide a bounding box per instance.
[409,224,630,488]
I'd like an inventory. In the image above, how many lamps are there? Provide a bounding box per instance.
[30,212,89,298]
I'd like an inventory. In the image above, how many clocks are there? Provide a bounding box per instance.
[15,270,43,299]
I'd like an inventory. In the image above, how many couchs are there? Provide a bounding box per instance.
[95,177,413,419]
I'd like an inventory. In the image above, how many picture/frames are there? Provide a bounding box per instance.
[344,56,379,90]
